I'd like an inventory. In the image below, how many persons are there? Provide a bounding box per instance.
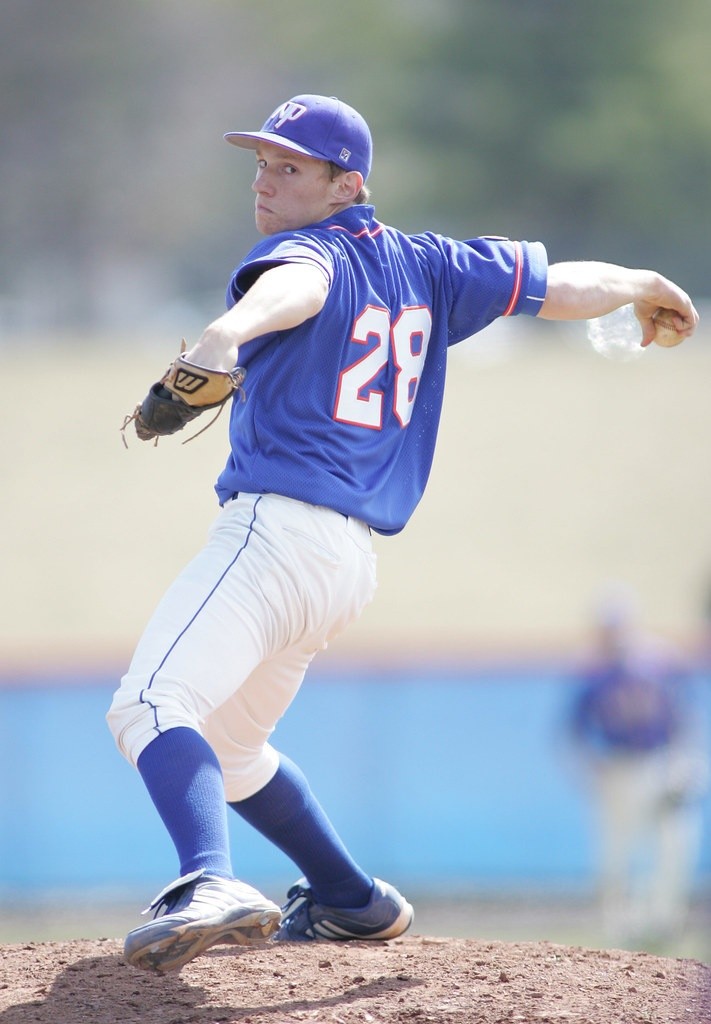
[557,591,695,950]
[103,94,701,972]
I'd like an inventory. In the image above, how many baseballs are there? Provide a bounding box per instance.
[650,308,686,347]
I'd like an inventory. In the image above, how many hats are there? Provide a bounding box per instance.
[224,94,372,186]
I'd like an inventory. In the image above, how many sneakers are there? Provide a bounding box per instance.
[125,868,282,972]
[272,877,413,941]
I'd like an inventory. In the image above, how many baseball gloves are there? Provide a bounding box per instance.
[121,339,246,448]
